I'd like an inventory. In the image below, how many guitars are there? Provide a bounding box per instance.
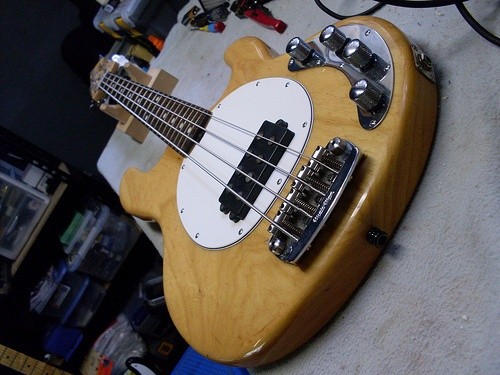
[84,14,439,370]
[0,345,171,375]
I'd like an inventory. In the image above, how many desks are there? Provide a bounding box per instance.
[97,0,500,375]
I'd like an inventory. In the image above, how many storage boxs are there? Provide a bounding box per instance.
[66,201,134,280]
[19,258,88,325]
[0,160,50,260]
